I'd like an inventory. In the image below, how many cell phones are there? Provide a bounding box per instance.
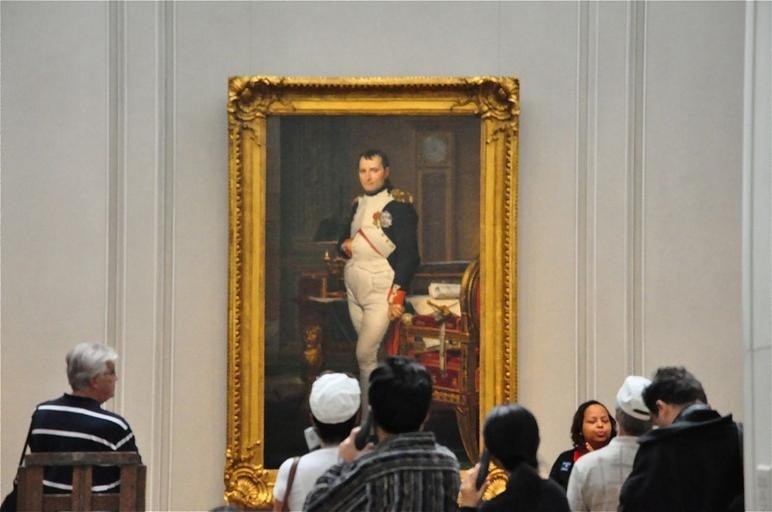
[356,405,373,450]
[476,448,490,490]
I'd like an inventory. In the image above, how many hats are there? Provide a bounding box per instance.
[309,370,362,425]
[617,373,654,423]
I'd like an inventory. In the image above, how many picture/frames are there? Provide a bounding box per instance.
[225,75,518,511]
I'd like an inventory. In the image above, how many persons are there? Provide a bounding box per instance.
[265,369,365,512]
[19,340,145,498]
[454,401,574,512]
[298,352,463,512]
[544,399,618,496]
[612,363,748,512]
[335,147,424,448]
[561,372,658,511]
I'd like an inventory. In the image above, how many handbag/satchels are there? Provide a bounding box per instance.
[0,472,21,512]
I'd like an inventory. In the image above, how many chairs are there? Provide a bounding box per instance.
[18,452,148,509]
